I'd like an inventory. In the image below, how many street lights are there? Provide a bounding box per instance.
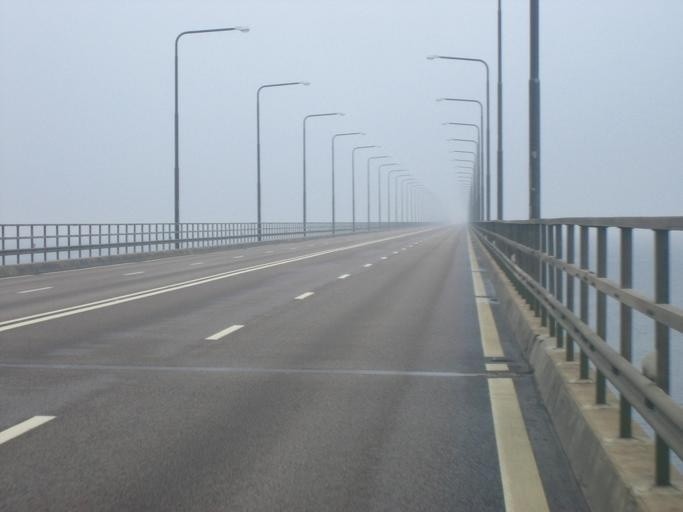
[332,132,367,236]
[426,55,491,222]
[367,155,390,229]
[256,81,310,242]
[377,162,425,230]
[352,145,378,232]
[303,112,346,236]
[173,25,251,253]
[441,122,481,221]
[437,97,484,220]
